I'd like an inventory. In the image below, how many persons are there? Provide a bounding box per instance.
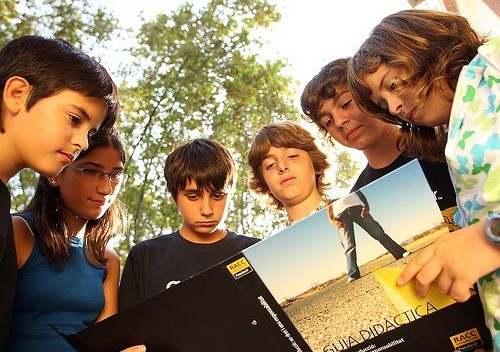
[348,8,500,352]
[247,121,341,225]
[0,34,148,352]
[327,190,411,282]
[301,58,461,234]
[119,138,262,315]
[13,126,126,352]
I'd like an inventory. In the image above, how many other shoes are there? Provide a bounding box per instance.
[403,252,411,258]
[347,277,355,284]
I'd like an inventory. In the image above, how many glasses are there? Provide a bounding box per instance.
[69,165,130,183]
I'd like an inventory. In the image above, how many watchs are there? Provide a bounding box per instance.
[485,214,500,244]
